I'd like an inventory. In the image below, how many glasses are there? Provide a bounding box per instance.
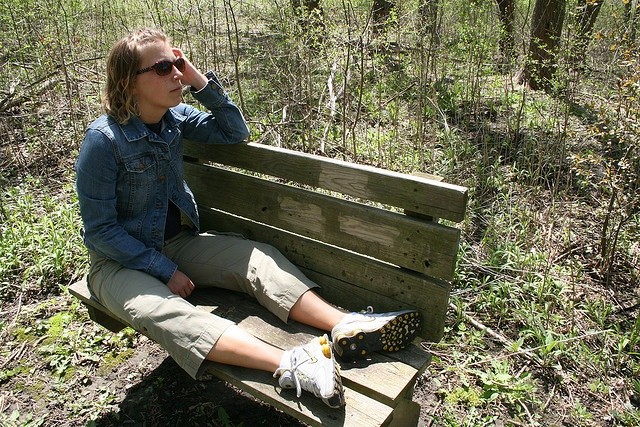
[136,58,185,76]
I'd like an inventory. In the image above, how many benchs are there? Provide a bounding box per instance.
[68,139,469,427]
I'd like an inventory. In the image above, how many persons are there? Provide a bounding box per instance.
[75,28,423,408]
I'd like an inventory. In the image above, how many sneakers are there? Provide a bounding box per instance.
[272,333,346,408]
[331,305,424,362]
